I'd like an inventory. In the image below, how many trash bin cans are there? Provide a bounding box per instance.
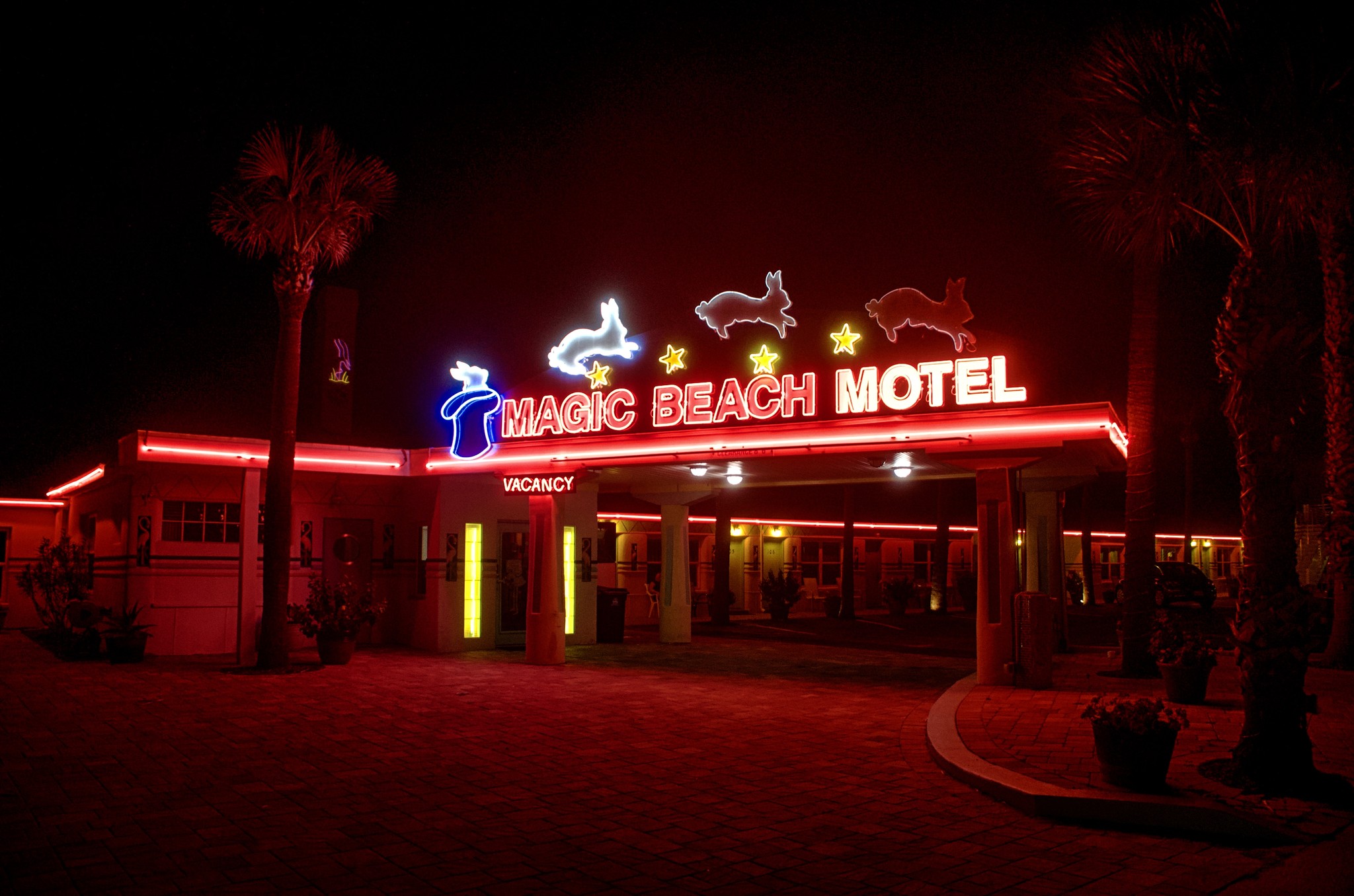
[596,585,630,643]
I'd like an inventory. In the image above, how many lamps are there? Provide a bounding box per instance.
[868,457,887,468]
[891,467,914,478]
[689,465,746,485]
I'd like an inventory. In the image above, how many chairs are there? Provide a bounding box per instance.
[913,577,960,608]
[802,578,824,612]
[645,584,660,618]
[836,577,862,611]
[690,582,711,617]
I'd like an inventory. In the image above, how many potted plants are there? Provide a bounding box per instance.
[1081,691,1190,790]
[878,575,914,615]
[1102,587,1116,604]
[1226,575,1240,599]
[822,588,841,617]
[955,571,977,610]
[287,571,390,664]
[96,599,159,664]
[759,566,800,619]
[1065,568,1084,605]
[1150,612,1218,704]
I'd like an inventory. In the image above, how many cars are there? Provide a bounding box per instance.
[1114,560,1217,610]
